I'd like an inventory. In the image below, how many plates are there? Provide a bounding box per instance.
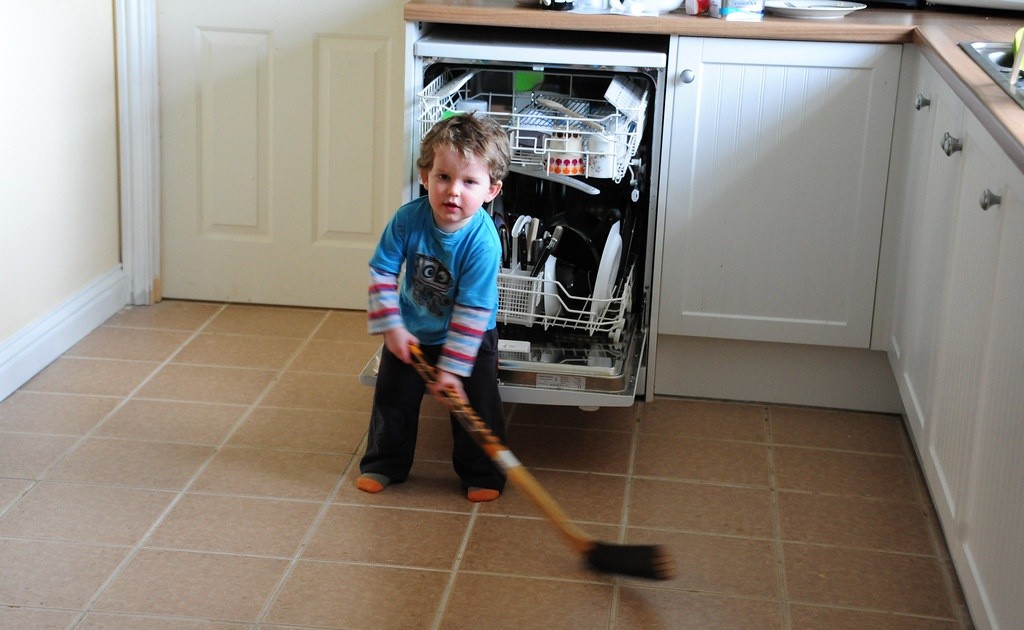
[590,221,623,322]
[764,1,867,19]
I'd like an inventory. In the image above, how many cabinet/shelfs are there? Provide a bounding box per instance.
[663,36,904,354]
[357,61,664,411]
[888,55,1024,630]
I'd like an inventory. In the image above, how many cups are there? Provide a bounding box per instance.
[546,130,585,176]
[586,133,619,178]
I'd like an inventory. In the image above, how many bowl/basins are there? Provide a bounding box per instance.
[543,224,598,321]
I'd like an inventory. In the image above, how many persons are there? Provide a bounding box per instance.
[355,110,511,501]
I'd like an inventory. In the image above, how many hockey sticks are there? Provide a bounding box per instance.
[407,343,679,582]
